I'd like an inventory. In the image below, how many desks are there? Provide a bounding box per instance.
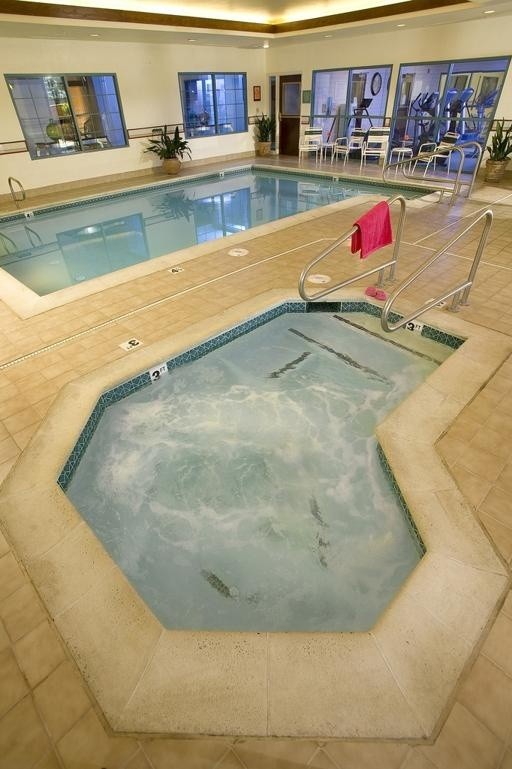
[35,136,108,157]
[186,123,234,137]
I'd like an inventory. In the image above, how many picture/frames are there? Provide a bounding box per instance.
[303,90,310,103]
[253,85,261,101]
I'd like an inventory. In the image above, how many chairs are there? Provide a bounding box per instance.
[298,126,460,177]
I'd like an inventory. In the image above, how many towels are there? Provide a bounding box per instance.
[351,201,393,259]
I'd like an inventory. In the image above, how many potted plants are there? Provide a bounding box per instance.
[144,123,192,175]
[485,116,512,183]
[255,111,276,155]
[152,190,196,222]
